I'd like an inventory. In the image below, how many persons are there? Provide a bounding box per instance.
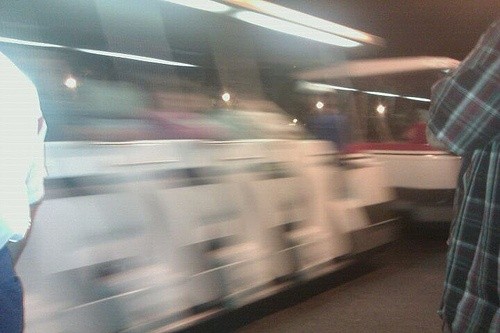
[0,51,48,333]
[52,84,433,148]
[423,15,500,333]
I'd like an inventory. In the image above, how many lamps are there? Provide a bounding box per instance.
[161,0,387,48]
